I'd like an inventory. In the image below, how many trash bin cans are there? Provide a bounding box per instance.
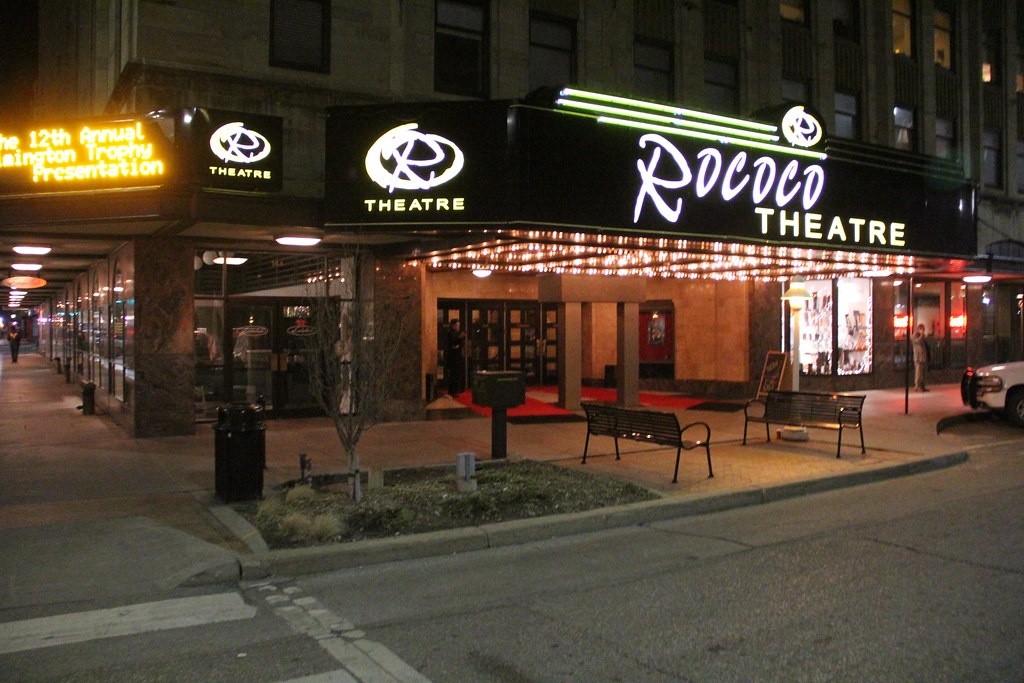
[211,401,269,504]
[604,364,618,388]
[426,373,434,401]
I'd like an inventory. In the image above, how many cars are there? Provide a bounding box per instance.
[961,361,1024,429]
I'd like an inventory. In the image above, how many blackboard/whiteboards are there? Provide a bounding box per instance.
[758,351,787,396]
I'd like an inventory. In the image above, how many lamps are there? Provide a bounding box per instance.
[194,250,234,270]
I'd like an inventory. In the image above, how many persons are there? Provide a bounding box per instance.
[909,324,933,392]
[444,318,467,397]
[7,325,21,362]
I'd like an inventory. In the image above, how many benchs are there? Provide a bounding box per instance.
[742,390,867,458]
[580,400,714,483]
